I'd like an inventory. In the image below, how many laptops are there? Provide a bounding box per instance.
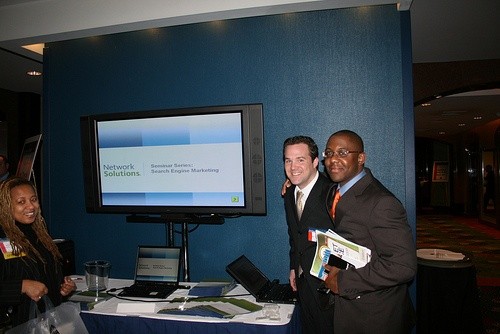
[117,245,182,299]
[226,255,298,304]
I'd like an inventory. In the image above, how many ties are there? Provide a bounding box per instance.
[330,191,341,220]
[295,191,304,220]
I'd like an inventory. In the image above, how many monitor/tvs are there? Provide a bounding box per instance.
[79,104,266,215]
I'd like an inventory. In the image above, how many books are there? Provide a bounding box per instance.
[310,228,371,299]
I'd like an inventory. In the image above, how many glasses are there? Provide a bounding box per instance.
[322,150,362,157]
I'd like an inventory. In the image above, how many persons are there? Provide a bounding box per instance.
[0,177,77,334]
[284,135,330,334]
[0,154,11,183]
[484,164,496,209]
[281,129,417,334]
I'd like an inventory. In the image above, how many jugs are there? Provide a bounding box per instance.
[83,259,109,292]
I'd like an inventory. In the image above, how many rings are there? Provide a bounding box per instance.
[39,296,41,299]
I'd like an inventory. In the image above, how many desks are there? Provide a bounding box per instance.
[67,274,301,334]
[416,247,478,334]
